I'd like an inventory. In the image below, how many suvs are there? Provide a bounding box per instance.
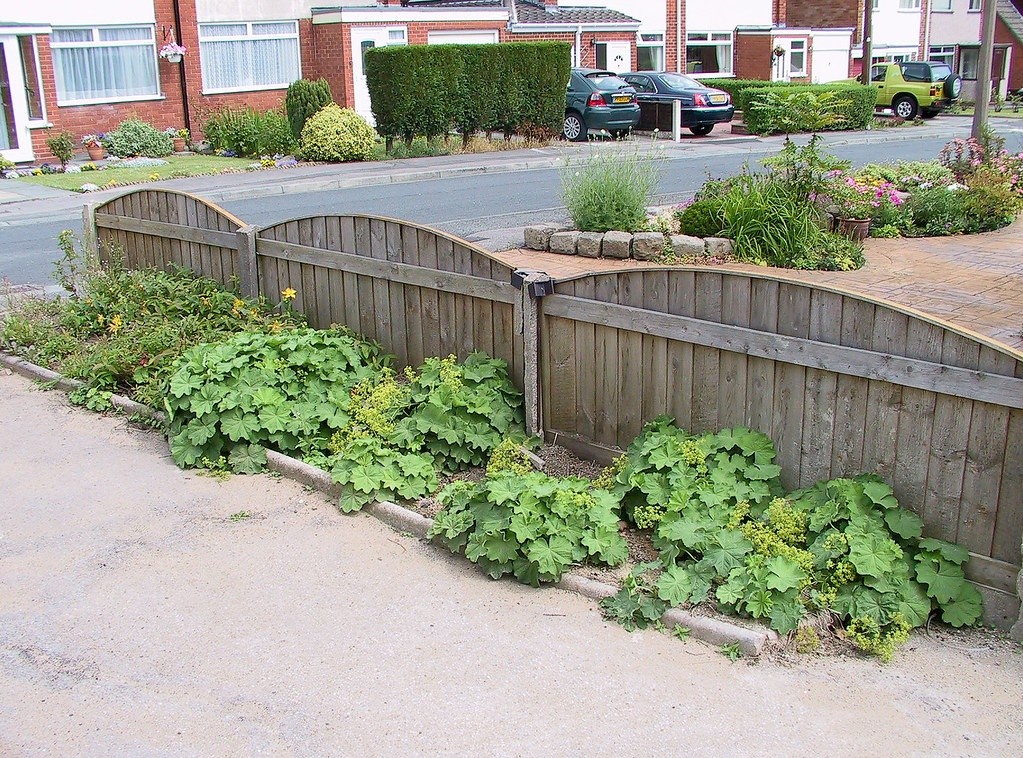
[562,67,641,142]
[848,60,962,122]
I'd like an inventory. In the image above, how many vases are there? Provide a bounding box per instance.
[166,53,183,63]
[775,51,783,56]
[173,137,187,152]
[86,146,105,160]
[835,216,872,242]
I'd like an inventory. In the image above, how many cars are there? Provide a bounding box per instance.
[617,71,735,137]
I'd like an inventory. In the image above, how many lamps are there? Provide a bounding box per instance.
[808,45,813,53]
[590,39,595,47]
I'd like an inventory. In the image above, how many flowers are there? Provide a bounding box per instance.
[81,131,111,148]
[807,169,902,221]
[166,127,189,139]
[158,41,186,59]
[773,46,786,56]
